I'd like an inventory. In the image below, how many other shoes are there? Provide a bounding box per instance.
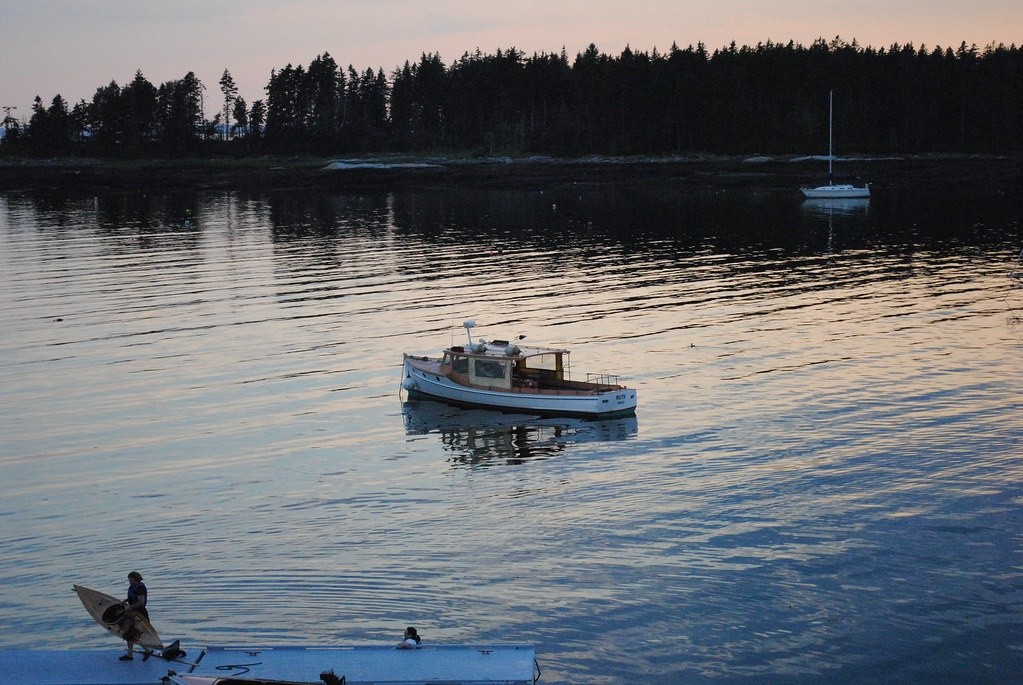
[143,649,153,660]
[118,654,135,660]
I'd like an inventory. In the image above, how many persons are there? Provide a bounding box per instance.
[118,571,155,662]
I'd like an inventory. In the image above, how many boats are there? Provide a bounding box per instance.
[168,672,304,685]
[71,583,163,652]
[798,90,873,198]
[398,320,638,422]
